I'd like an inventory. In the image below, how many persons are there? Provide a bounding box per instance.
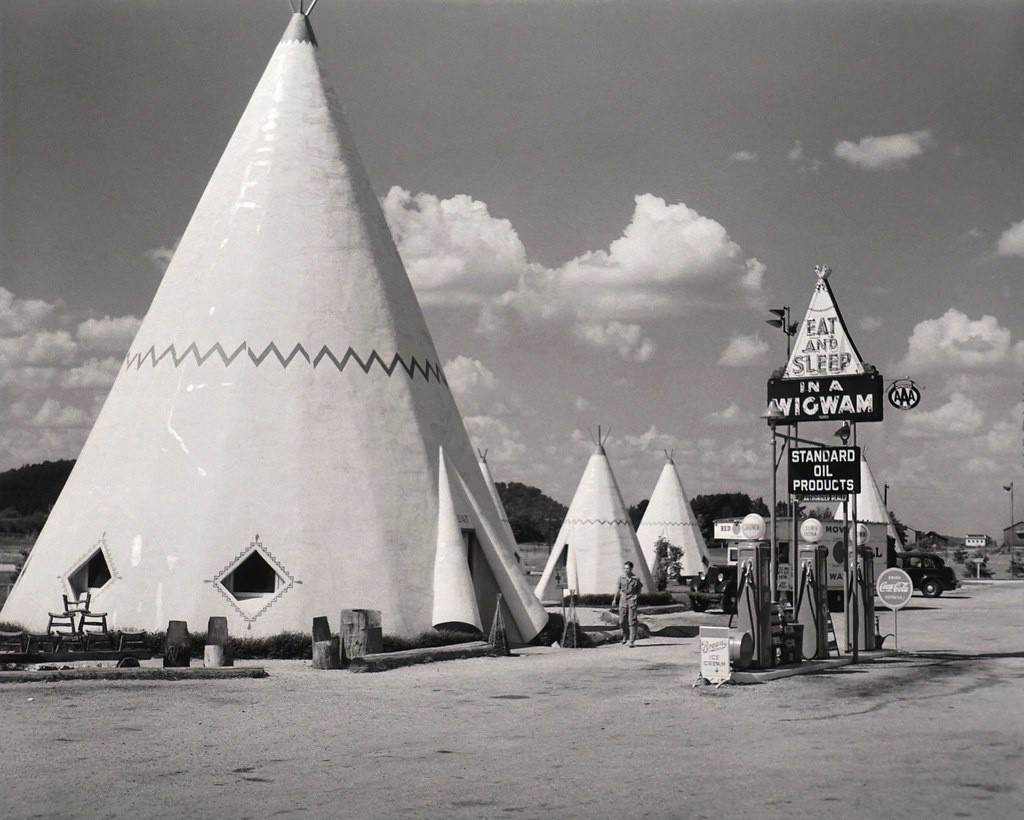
[614,560,643,644]
[9,548,29,584]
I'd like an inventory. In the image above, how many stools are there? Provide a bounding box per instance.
[63,593,93,615]
[45,612,76,634]
[86,630,116,652]
[0,630,26,654]
[55,630,85,652]
[77,612,108,633]
[118,631,148,649]
[26,632,55,655]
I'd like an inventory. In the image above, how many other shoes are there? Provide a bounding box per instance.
[628,642,634,647]
[622,637,626,644]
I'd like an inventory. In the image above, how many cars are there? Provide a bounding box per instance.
[895,549,963,597]
[688,562,738,614]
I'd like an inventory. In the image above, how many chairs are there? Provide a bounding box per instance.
[311,616,342,670]
[205,616,229,668]
[163,620,193,668]
